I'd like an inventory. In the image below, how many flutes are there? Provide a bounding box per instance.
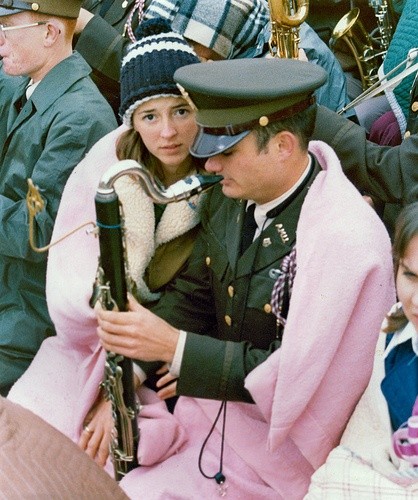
[95,158,225,484]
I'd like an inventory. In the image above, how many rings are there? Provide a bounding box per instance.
[86,427,93,432]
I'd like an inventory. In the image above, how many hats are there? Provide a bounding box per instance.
[0,0,81,18]
[118,19,200,128]
[173,59,328,157]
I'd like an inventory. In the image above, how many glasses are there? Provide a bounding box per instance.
[0,21,61,38]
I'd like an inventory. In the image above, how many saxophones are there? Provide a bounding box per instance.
[268,0,309,64]
[331,0,399,95]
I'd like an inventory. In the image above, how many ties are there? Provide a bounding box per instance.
[240,203,258,257]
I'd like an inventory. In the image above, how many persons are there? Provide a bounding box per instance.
[0,0,418,500]
